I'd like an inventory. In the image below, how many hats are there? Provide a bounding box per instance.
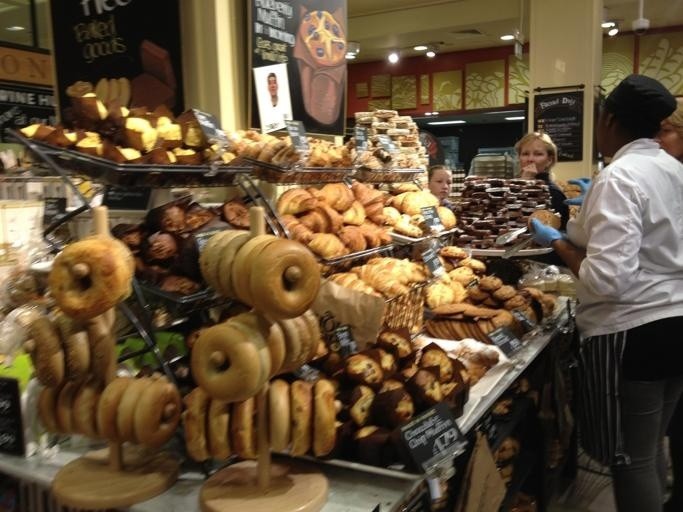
[603,73,678,133]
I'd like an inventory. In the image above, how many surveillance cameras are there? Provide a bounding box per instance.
[633,18,650,36]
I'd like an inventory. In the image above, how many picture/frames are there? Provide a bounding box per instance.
[247,0,348,138]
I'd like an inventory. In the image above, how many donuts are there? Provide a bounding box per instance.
[28,229,338,462]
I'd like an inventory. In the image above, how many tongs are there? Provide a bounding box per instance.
[497,211,561,260]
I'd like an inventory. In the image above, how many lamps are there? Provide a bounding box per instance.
[632,0,650,36]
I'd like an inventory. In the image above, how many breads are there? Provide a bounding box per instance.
[109,179,581,461]
[20,93,430,175]
[67,41,177,112]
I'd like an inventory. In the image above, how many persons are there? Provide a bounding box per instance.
[510,130,569,234]
[654,96,683,161]
[427,163,460,217]
[525,74,682,511]
[263,73,286,129]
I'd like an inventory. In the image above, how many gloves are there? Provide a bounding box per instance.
[529,217,568,249]
[562,177,592,207]
